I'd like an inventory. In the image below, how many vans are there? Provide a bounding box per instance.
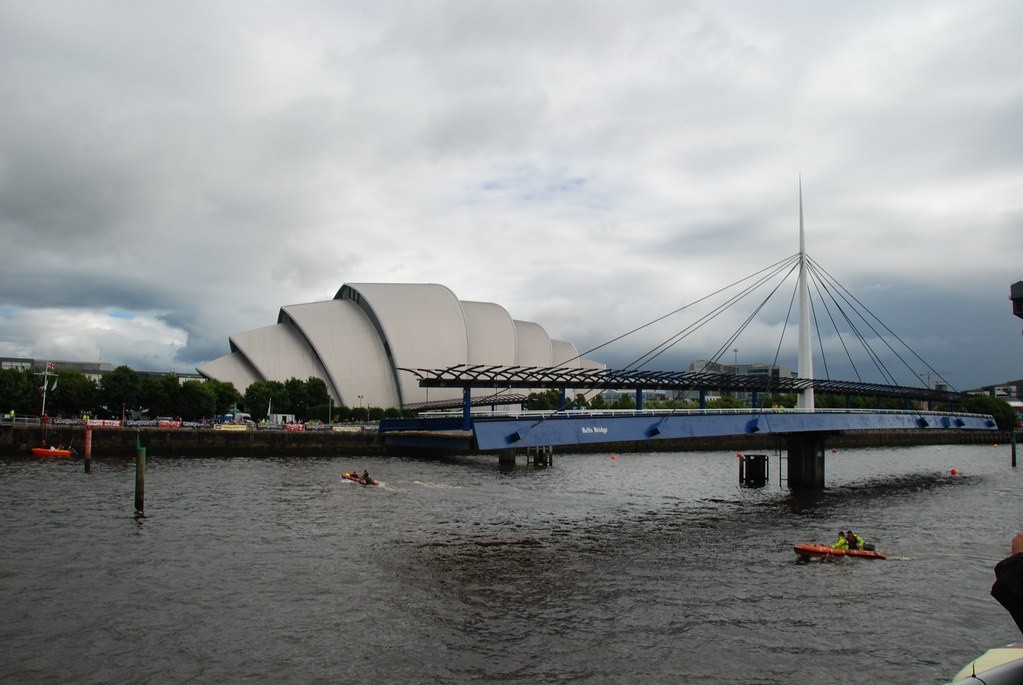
[235,413,252,425]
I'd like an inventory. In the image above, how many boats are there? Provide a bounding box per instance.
[793,543,887,560]
[342,471,379,487]
[32,446,73,458]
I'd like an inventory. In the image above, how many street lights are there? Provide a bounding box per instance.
[359,395,363,410]
[329,394,334,422]
[734,348,740,397]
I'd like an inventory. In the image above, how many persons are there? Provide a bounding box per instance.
[255,417,323,430]
[828,530,863,551]
[991,534,1023,635]
[42,441,65,452]
[11,409,15,422]
[350,469,369,481]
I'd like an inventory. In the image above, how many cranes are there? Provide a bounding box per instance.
[920,369,952,389]
